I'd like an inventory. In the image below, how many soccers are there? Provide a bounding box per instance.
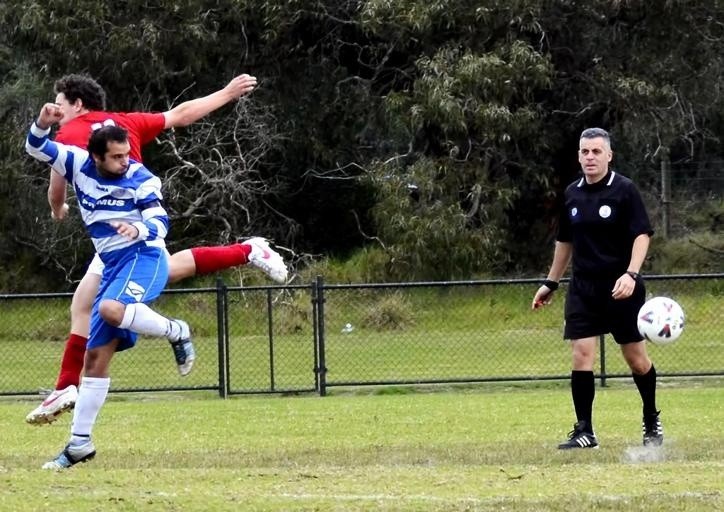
[637,296,684,344]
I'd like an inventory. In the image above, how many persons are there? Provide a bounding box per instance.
[23,73,289,427]
[22,102,197,471]
[530,126,664,451]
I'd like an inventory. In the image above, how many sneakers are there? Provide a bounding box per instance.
[241,236,287,284]
[42,440,96,469]
[25,385,78,423]
[643,410,663,447]
[559,422,599,450]
[167,319,195,376]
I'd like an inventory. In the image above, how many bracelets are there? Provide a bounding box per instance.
[626,271,640,282]
[543,279,558,292]
